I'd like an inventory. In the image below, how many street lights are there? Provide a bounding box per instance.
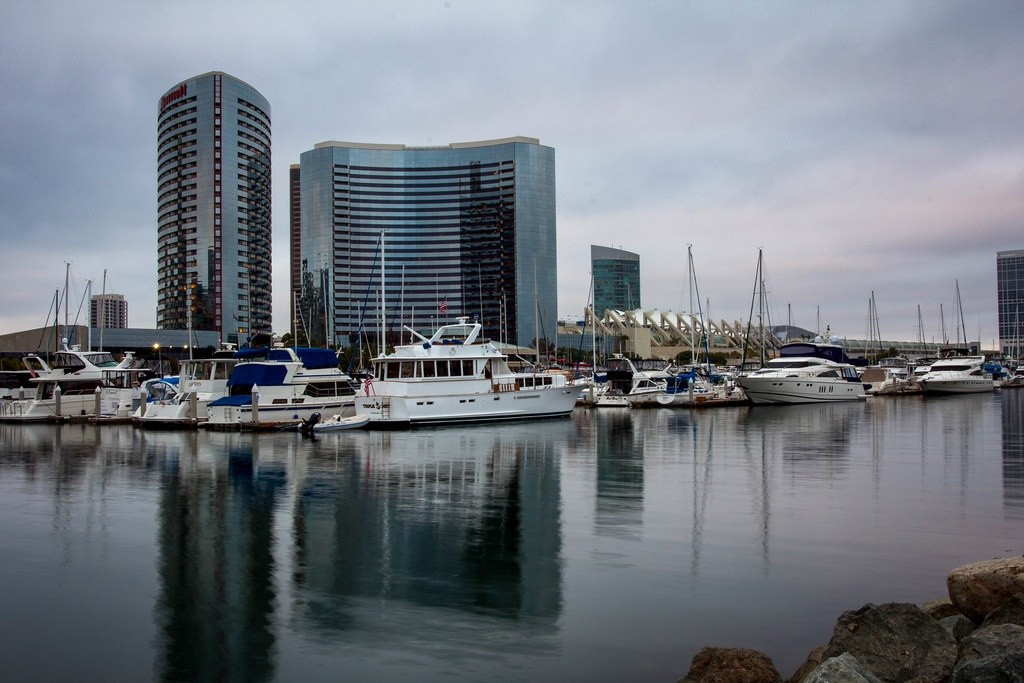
[154,343,163,379]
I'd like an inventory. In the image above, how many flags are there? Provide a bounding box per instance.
[440,296,446,312]
[365,375,372,398]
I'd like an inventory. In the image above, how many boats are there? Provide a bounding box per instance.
[980,355,1024,387]
[131,358,247,429]
[53,345,164,387]
[198,361,357,430]
[355,316,587,428]
[855,357,941,395]
[297,414,371,434]
[0,338,138,422]
[734,341,874,405]
[0,369,71,415]
[88,367,156,422]
[595,353,667,407]
[915,354,995,394]
[541,366,608,403]
[656,246,763,406]
